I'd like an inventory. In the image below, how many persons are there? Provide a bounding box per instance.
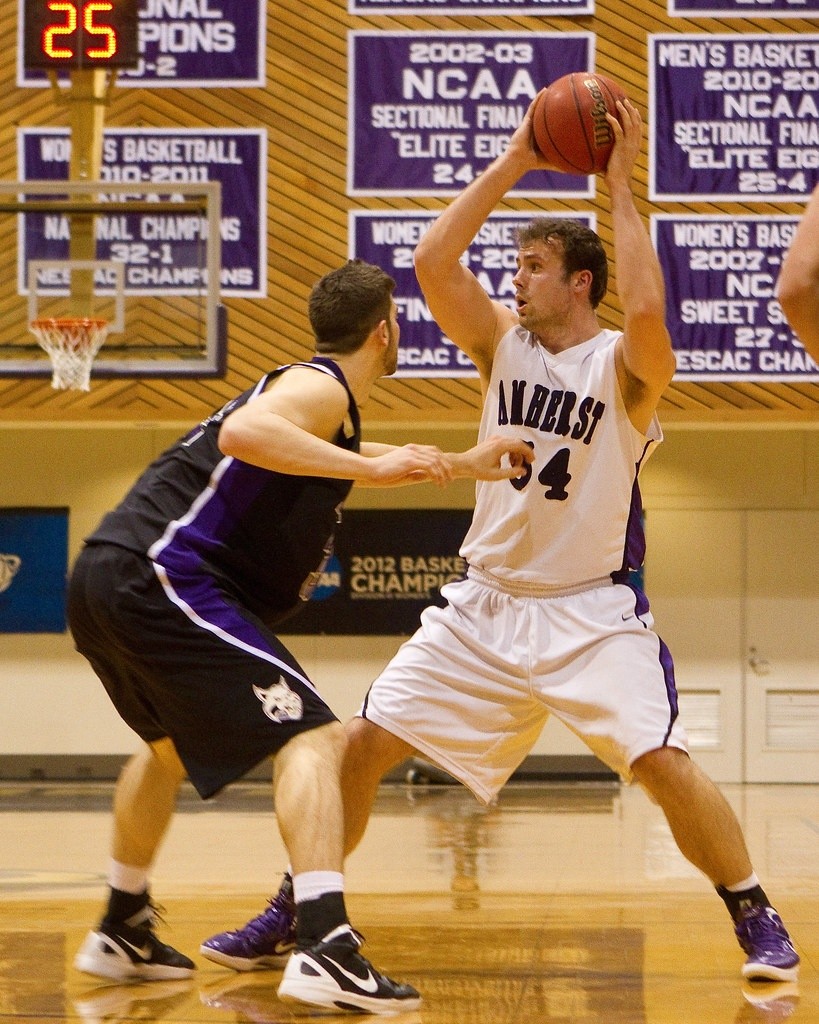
[777,180,819,370]
[200,88,800,982]
[65,261,535,1014]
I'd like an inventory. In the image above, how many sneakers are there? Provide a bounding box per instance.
[75,927,195,981]
[731,907,800,981]
[276,926,423,1015]
[198,873,298,971]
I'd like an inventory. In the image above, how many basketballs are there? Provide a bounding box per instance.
[532,72,629,175]
